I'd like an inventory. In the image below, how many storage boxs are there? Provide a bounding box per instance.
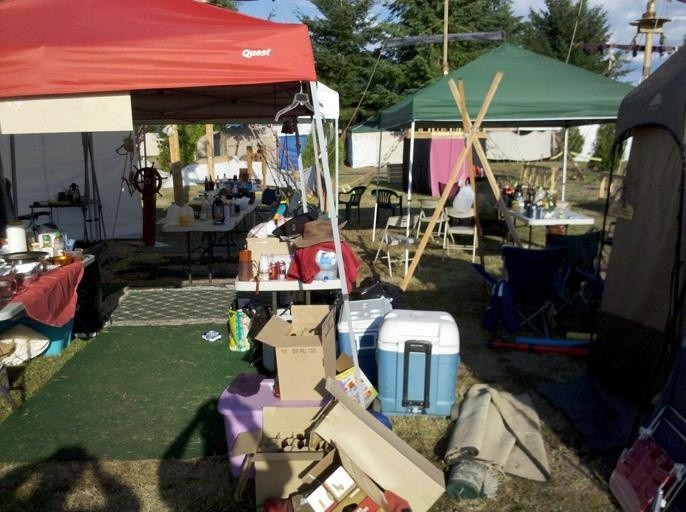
[217,300,338,508]
[336,297,393,384]
[374,308,461,418]
[17,304,82,358]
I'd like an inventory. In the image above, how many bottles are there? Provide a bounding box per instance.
[42,235,50,248]
[237,249,251,281]
[501,182,534,211]
[201,173,253,226]
[53,230,65,249]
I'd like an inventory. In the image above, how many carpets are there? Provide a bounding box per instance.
[0,324,264,464]
[106,286,237,325]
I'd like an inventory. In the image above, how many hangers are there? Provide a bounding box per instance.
[274,81,328,123]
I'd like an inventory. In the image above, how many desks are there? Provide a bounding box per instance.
[494,202,595,250]
[234,275,343,368]
[0,254,96,414]
[29,199,102,243]
[161,199,261,286]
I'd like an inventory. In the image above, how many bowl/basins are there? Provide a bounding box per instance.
[4,252,73,266]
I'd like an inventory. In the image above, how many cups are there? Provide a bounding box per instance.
[0,276,16,301]
[190,204,202,221]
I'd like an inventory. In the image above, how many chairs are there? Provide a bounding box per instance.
[473,230,602,339]
[337,186,480,278]
